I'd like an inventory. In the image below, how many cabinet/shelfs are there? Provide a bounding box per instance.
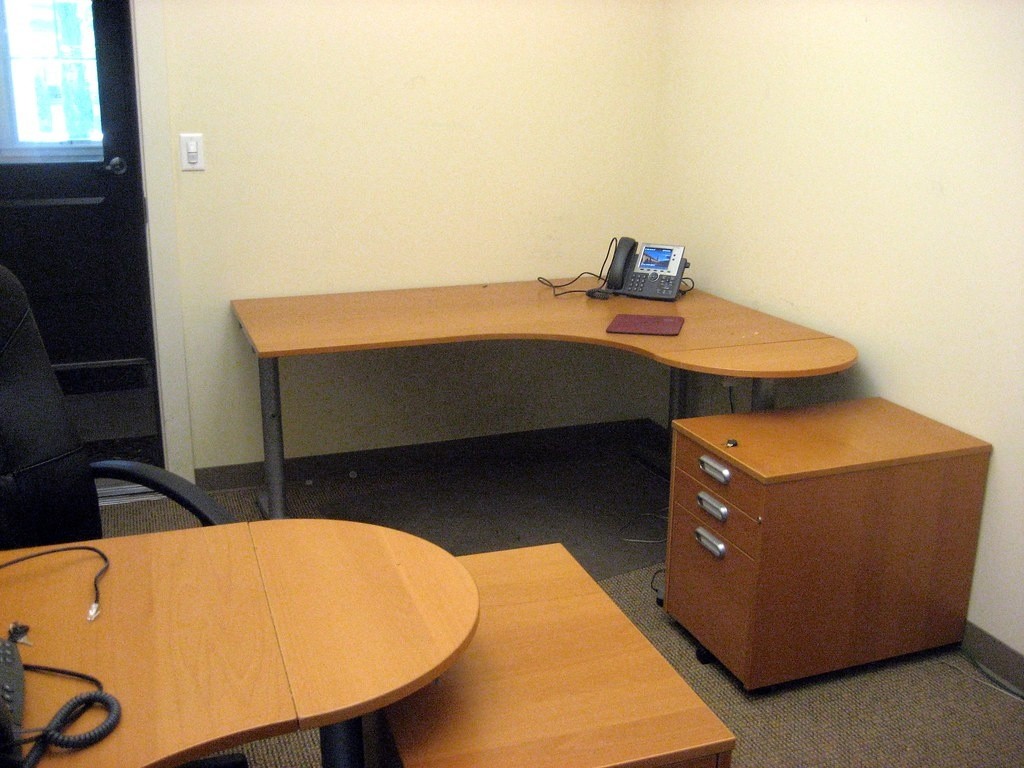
[396,544,735,768]
[667,398,994,687]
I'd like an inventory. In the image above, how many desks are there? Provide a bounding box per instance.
[0,518,481,768]
[234,279,860,522]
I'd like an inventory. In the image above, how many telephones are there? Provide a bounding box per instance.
[605,237,686,300]
[0,639,26,768]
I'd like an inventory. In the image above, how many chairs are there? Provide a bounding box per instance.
[1,266,247,768]
